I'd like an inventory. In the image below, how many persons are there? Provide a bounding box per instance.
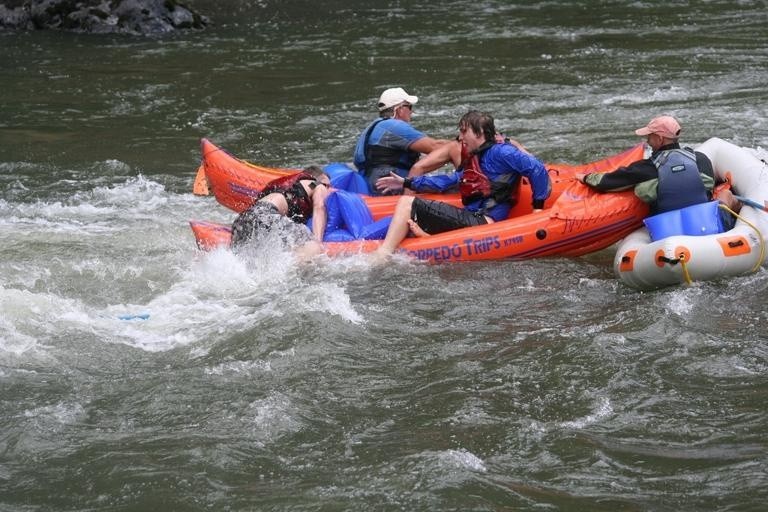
[403,128,537,184]
[351,86,457,194]
[230,163,377,261]
[362,110,552,268]
[567,114,743,235]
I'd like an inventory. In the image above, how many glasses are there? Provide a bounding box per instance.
[402,103,412,111]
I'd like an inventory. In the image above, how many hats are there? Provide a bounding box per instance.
[377,86,419,112]
[634,116,681,139]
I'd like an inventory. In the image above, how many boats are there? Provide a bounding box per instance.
[187,138,646,216]
[613,136,768,293]
[187,183,651,269]
